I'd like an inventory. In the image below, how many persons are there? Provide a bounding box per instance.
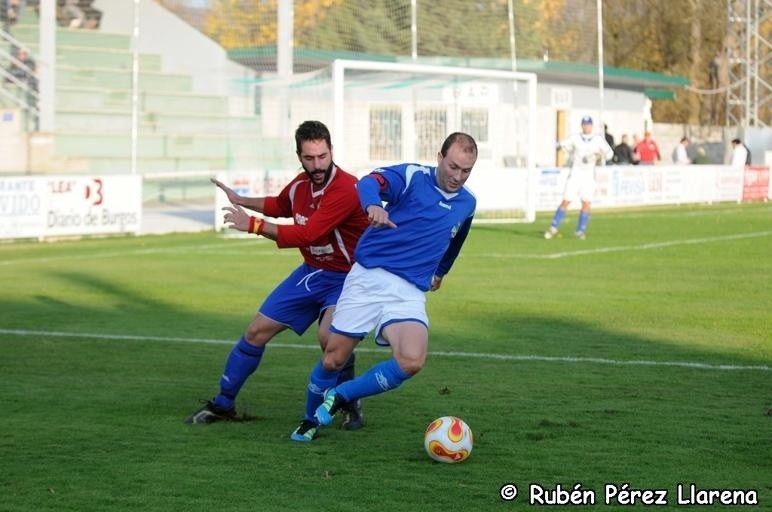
[709,50,721,89]
[288,133,480,443]
[601,122,661,165]
[186,120,373,429]
[1,0,104,29]
[695,149,713,164]
[6,47,36,82]
[742,144,753,166]
[729,139,748,166]
[671,137,691,165]
[543,115,615,240]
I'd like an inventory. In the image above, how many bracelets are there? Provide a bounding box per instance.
[248,216,265,236]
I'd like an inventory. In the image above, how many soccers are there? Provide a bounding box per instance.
[424,416,473,464]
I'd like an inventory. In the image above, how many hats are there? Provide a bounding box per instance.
[581,115,593,128]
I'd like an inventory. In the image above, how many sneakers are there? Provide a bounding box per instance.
[544,225,558,239]
[339,399,365,431]
[290,418,320,442]
[184,396,242,425]
[314,387,347,426]
[576,230,586,240]
